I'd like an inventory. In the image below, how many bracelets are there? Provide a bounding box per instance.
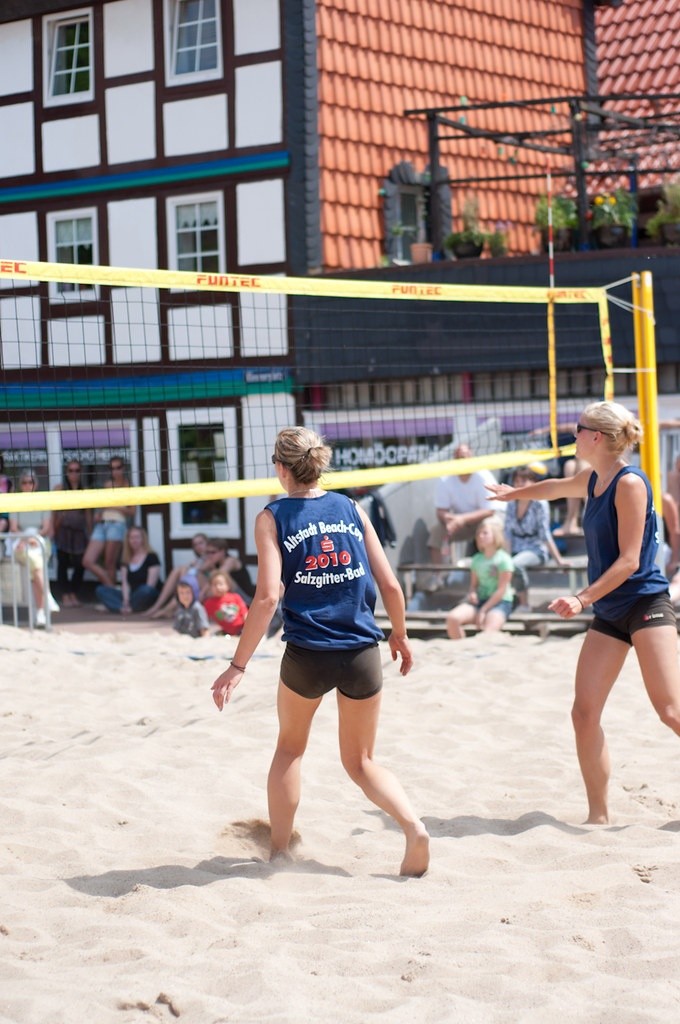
[230,661,246,673]
[575,596,585,613]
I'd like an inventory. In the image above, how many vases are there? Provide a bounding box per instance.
[589,224,628,250]
[491,250,508,259]
[410,243,433,263]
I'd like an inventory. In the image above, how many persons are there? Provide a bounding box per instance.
[95,526,166,614]
[446,516,515,639]
[504,465,570,619]
[0,454,9,542]
[138,533,286,638]
[417,399,680,825]
[51,458,92,608]
[82,456,137,612]
[7,468,60,628]
[210,426,431,878]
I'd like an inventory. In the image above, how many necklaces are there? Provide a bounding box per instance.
[289,488,322,496]
[599,458,622,487]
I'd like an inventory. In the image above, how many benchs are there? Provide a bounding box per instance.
[373,497,595,641]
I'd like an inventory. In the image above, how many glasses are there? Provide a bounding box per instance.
[272,454,293,468]
[21,480,33,484]
[110,466,123,470]
[206,548,222,555]
[68,468,81,473]
[577,421,615,438]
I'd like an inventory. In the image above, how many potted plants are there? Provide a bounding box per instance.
[443,199,492,261]
[532,196,580,253]
[643,180,680,246]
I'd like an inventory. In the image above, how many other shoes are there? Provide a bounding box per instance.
[36,607,48,628]
[46,593,60,613]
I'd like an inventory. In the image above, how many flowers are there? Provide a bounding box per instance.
[489,220,512,254]
[585,186,639,236]
